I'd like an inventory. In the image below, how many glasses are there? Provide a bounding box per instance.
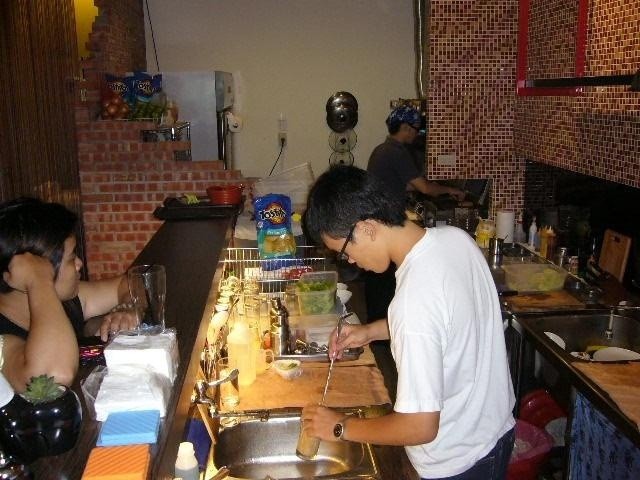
[336,218,361,263]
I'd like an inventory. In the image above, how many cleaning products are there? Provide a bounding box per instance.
[527,215,538,245]
[515,209,527,243]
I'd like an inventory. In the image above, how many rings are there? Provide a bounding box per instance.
[109,329,117,335]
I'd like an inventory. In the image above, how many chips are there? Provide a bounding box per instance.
[262,236,296,252]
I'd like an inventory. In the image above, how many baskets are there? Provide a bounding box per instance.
[220,245,329,305]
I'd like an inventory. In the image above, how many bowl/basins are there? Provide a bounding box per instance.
[207,185,242,205]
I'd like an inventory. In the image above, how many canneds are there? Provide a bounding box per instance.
[556,247,566,266]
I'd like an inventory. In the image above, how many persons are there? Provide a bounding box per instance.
[301,164,520,480]
[363,103,471,350]
[1,194,149,404]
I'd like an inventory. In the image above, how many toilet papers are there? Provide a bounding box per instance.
[228,115,243,133]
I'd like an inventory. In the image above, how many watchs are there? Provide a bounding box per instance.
[332,415,351,442]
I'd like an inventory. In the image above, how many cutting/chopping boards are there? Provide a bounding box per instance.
[508,290,587,313]
[598,229,632,283]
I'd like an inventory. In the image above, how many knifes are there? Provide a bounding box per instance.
[499,291,543,296]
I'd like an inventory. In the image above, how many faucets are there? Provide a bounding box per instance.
[188,379,220,419]
[603,303,640,340]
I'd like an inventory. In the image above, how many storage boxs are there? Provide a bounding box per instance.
[294,271,339,316]
[501,263,568,294]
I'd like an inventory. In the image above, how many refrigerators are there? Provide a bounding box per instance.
[126,70,233,169]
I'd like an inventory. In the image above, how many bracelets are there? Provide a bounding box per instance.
[111,302,146,319]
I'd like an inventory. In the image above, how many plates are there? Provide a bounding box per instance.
[593,346,640,361]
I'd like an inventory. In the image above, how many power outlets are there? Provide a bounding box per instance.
[278,132,288,146]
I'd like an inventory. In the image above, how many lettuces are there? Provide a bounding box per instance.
[297,275,337,316]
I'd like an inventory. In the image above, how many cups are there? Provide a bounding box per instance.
[296,402,329,460]
[217,356,240,408]
[489,238,504,255]
[256,349,274,375]
[128,265,166,336]
[244,284,269,318]
[274,359,302,380]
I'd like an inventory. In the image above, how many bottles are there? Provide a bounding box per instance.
[270,297,289,354]
[475,219,495,249]
[176,442,199,480]
[227,322,256,386]
[515,215,558,259]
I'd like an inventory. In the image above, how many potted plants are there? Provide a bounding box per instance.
[19,373,83,457]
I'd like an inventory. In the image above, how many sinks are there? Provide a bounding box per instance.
[530,311,640,362]
[208,408,381,478]
